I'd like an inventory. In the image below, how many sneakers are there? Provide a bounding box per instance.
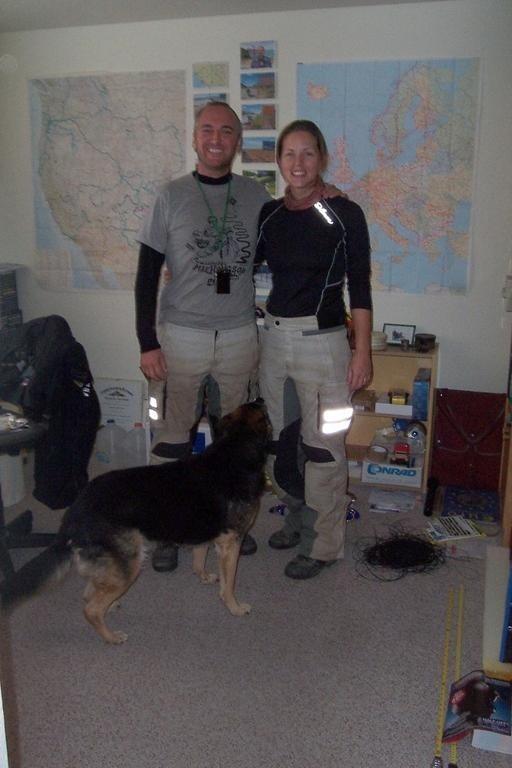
[285,554,337,579]
[268,530,302,549]
[152,539,182,572]
[239,533,257,556]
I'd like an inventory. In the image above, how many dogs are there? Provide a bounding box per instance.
[56,396,273,644]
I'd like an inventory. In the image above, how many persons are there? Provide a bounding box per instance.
[249,119,374,580]
[250,45,273,68]
[134,100,349,574]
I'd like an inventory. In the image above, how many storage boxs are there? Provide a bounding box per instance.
[373,389,414,416]
[359,428,425,490]
[411,364,432,421]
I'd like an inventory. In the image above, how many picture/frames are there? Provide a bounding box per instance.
[382,322,416,347]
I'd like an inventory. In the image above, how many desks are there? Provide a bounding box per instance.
[1,400,69,607]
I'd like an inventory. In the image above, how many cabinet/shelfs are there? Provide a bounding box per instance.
[342,337,443,519]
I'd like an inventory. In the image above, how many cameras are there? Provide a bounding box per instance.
[215,270,231,294]
